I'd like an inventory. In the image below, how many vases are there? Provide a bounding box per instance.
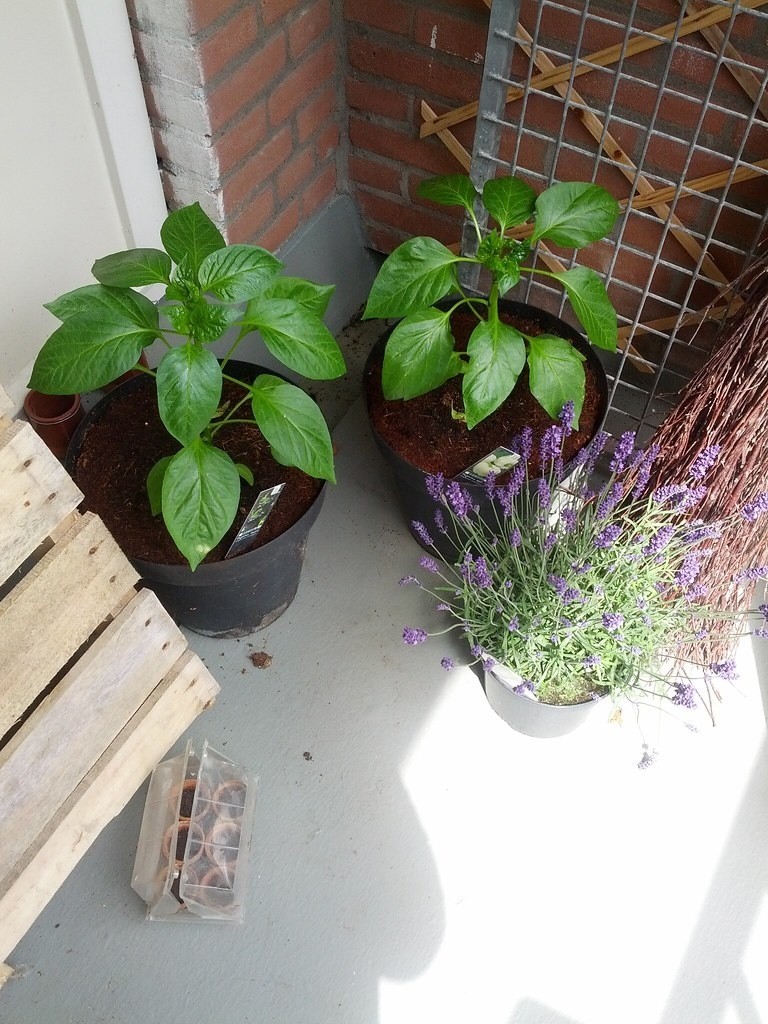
[479,655,614,739]
[200,866,236,911]
[212,780,248,822]
[163,820,205,865]
[169,779,211,822]
[155,864,199,909]
[203,823,243,867]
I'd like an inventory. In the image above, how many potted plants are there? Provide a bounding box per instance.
[27,203,347,639]
[358,173,626,567]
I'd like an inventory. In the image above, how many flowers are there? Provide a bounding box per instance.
[400,401,768,770]
